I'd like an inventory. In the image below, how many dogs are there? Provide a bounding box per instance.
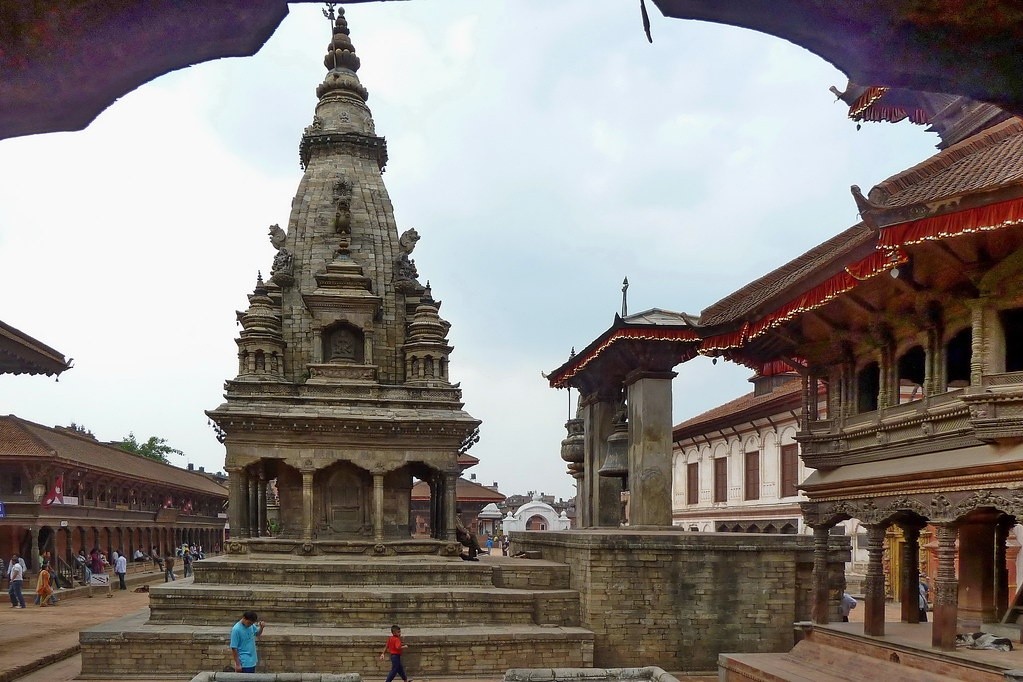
[130,585,149,593]
[955,632,1013,651]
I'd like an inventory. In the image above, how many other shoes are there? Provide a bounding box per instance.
[404,679,413,682]
[468,551,490,561]
[50,587,66,592]
[9,600,58,609]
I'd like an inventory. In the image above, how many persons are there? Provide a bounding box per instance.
[1,542,221,586]
[842,592,857,622]
[919,580,929,622]
[486,534,510,554]
[228,611,266,674]
[380,625,414,682]
[35,558,61,605]
[7,558,27,609]
[35,564,54,608]
[455,516,490,562]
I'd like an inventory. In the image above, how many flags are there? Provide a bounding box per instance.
[181,499,193,514]
[42,474,65,507]
[162,496,172,512]
